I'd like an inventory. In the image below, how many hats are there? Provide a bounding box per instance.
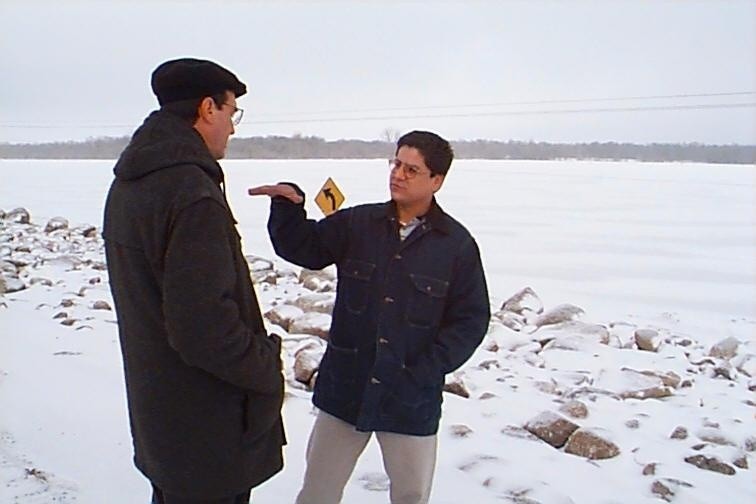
[151,57,248,109]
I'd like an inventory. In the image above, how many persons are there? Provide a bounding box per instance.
[101,58,286,504]
[247,130,492,504]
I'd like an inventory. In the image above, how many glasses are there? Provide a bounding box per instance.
[388,159,430,180]
[222,104,244,126]
